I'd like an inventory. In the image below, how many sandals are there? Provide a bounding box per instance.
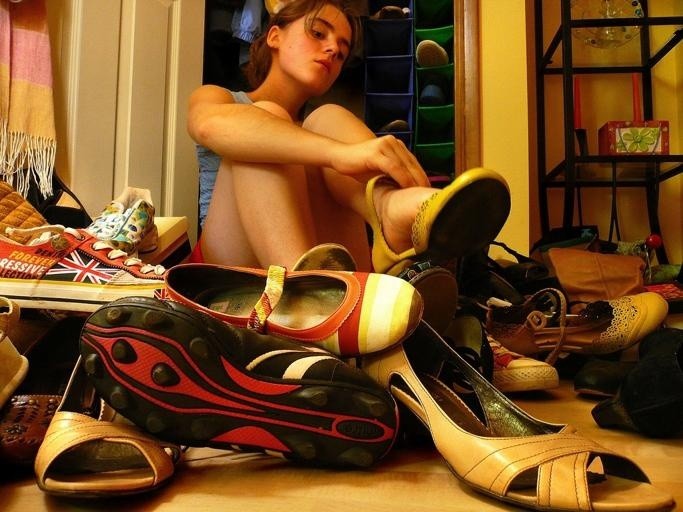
[379,120,408,131]
[1,167,683,511]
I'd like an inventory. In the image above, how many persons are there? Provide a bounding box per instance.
[183,0,511,275]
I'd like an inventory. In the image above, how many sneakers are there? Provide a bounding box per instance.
[416,40,449,67]
[421,85,444,106]
[379,6,404,19]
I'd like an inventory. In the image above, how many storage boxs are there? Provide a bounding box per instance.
[598,119,670,156]
[139,217,194,270]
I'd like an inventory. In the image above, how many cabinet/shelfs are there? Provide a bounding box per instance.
[364,1,457,178]
[535,0,683,266]
